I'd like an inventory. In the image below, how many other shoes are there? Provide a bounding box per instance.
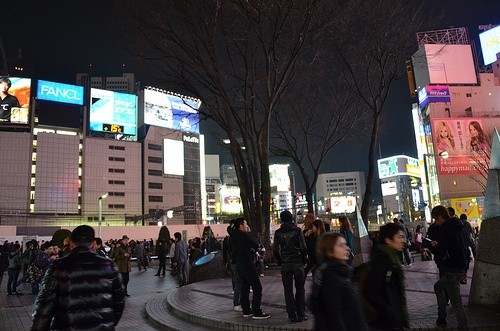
[5,289,18,295]
[290,316,299,322]
[401,263,412,267]
[297,315,308,322]
[234,305,252,312]
[435,318,447,328]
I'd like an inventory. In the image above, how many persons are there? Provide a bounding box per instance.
[339,216,355,265]
[393,217,426,266]
[0,77,20,119]
[436,121,455,152]
[272,210,308,322]
[30,225,125,331]
[467,121,488,151]
[93,230,220,298]
[310,232,369,331]
[222,218,271,319]
[303,219,331,276]
[431,205,470,331]
[0,237,72,294]
[367,223,409,331]
[447,206,479,259]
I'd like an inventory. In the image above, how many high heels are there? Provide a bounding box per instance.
[162,272,165,275]
[154,272,161,276]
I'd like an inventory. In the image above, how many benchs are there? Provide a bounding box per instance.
[403,249,423,263]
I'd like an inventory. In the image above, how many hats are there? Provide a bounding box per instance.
[121,235,129,240]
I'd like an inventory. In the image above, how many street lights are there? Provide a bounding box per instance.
[97,192,110,238]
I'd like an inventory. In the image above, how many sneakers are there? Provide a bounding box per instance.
[251,312,271,320]
[242,310,254,318]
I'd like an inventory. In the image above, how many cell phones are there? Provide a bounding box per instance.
[425,237,432,241]
